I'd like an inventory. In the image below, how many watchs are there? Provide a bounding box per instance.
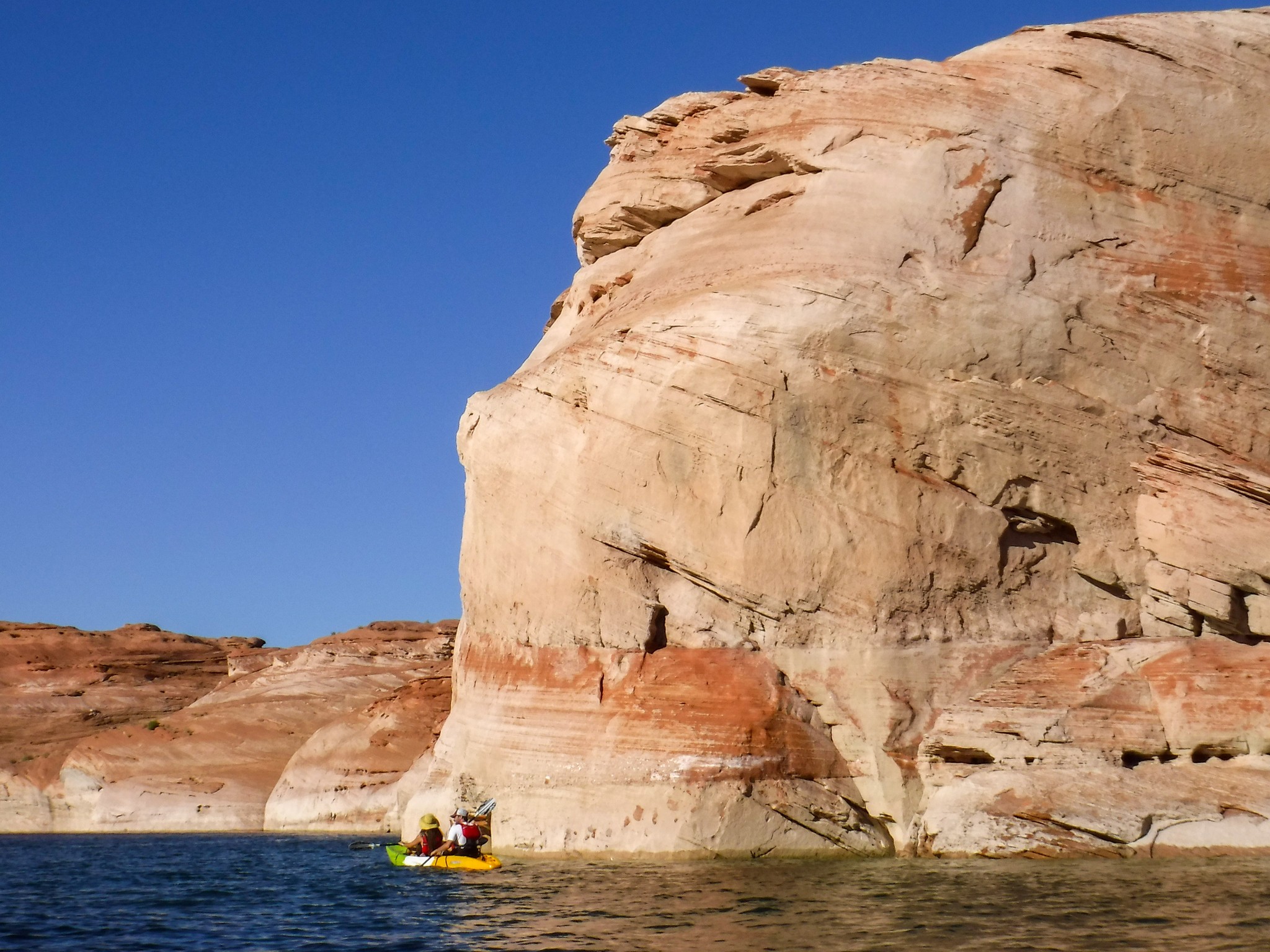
[401,843,405,846]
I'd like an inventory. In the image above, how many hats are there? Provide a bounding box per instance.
[450,808,469,818]
[419,813,440,830]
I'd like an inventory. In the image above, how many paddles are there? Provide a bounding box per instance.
[419,797,495,867]
[348,841,399,852]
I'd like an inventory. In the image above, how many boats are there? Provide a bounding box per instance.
[385,842,502,872]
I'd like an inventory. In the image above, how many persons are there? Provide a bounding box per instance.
[430,809,482,859]
[398,814,443,856]
